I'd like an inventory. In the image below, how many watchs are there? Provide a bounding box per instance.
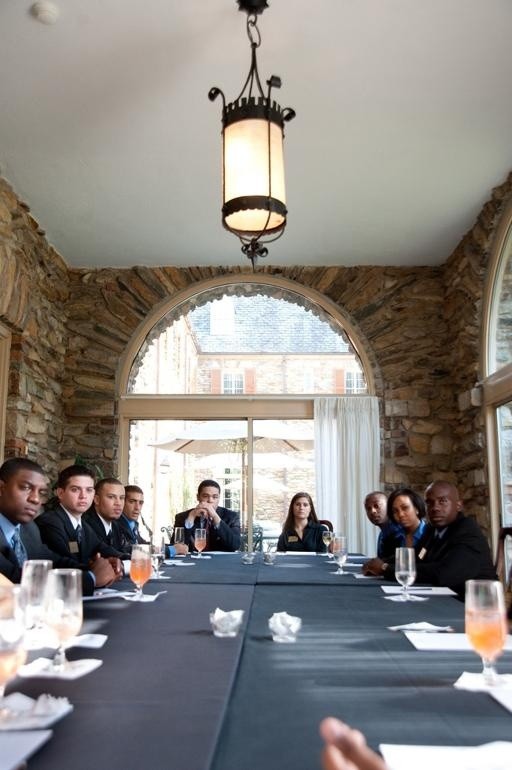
[382,563,389,570]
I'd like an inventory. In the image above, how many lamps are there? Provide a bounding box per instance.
[207,0,296,277]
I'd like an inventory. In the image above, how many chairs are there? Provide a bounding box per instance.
[319,520,333,534]
[494,527,512,600]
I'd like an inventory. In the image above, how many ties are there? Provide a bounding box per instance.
[12,529,28,568]
[75,524,82,543]
[108,530,114,545]
[203,518,210,534]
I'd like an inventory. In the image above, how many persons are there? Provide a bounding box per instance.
[363,489,430,576]
[277,492,328,552]
[368,480,500,603]
[320,716,386,770]
[364,492,401,558]
[171,480,241,552]
[81,478,132,560]
[34,465,132,577]
[118,486,189,558]
[0,458,123,596]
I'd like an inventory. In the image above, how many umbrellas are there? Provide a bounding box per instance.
[147,420,315,552]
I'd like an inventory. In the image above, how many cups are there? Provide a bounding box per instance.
[0,585,30,720]
[465,580,508,687]
[174,527,184,544]
[130,543,151,600]
[19,559,52,612]
[322,530,333,556]
[394,548,416,599]
[43,569,84,673]
[194,528,206,556]
[332,537,348,575]
